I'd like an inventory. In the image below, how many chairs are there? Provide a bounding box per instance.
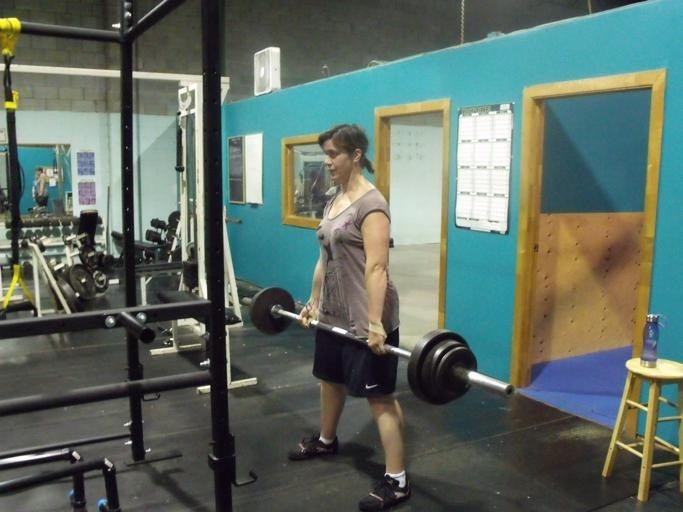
[287,432,339,459]
[359,474,412,511]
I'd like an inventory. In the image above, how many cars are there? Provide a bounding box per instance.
[640,313,659,369]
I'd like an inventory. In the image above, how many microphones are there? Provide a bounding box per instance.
[602,357,682,500]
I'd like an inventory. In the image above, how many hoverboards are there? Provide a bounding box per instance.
[242,287,514,404]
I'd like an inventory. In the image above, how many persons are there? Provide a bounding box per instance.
[286,124,411,512]
